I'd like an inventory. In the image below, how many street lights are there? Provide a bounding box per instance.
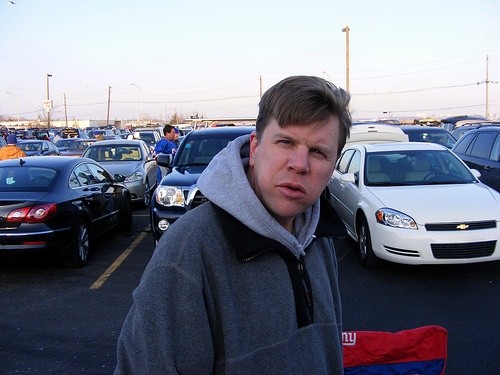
[106,84,113,126]
[46,73,53,133]
[130,83,143,121]
[341,25,351,94]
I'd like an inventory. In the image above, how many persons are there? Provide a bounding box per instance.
[0,135,27,161]
[98,132,105,141]
[0,131,9,147]
[53,131,63,144]
[41,135,50,141]
[113,128,120,135]
[412,119,422,127]
[153,124,180,186]
[23,128,28,137]
[114,76,352,375]
[80,129,90,139]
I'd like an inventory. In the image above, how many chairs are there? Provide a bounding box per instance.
[195,140,217,165]
[367,158,390,182]
[405,157,433,182]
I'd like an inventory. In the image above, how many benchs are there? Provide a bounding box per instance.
[384,160,409,181]
[6,172,51,187]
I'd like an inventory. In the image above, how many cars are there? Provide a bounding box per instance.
[149,124,260,250]
[322,141,500,268]
[6,123,259,213]
[344,112,500,194]
[0,155,134,270]
